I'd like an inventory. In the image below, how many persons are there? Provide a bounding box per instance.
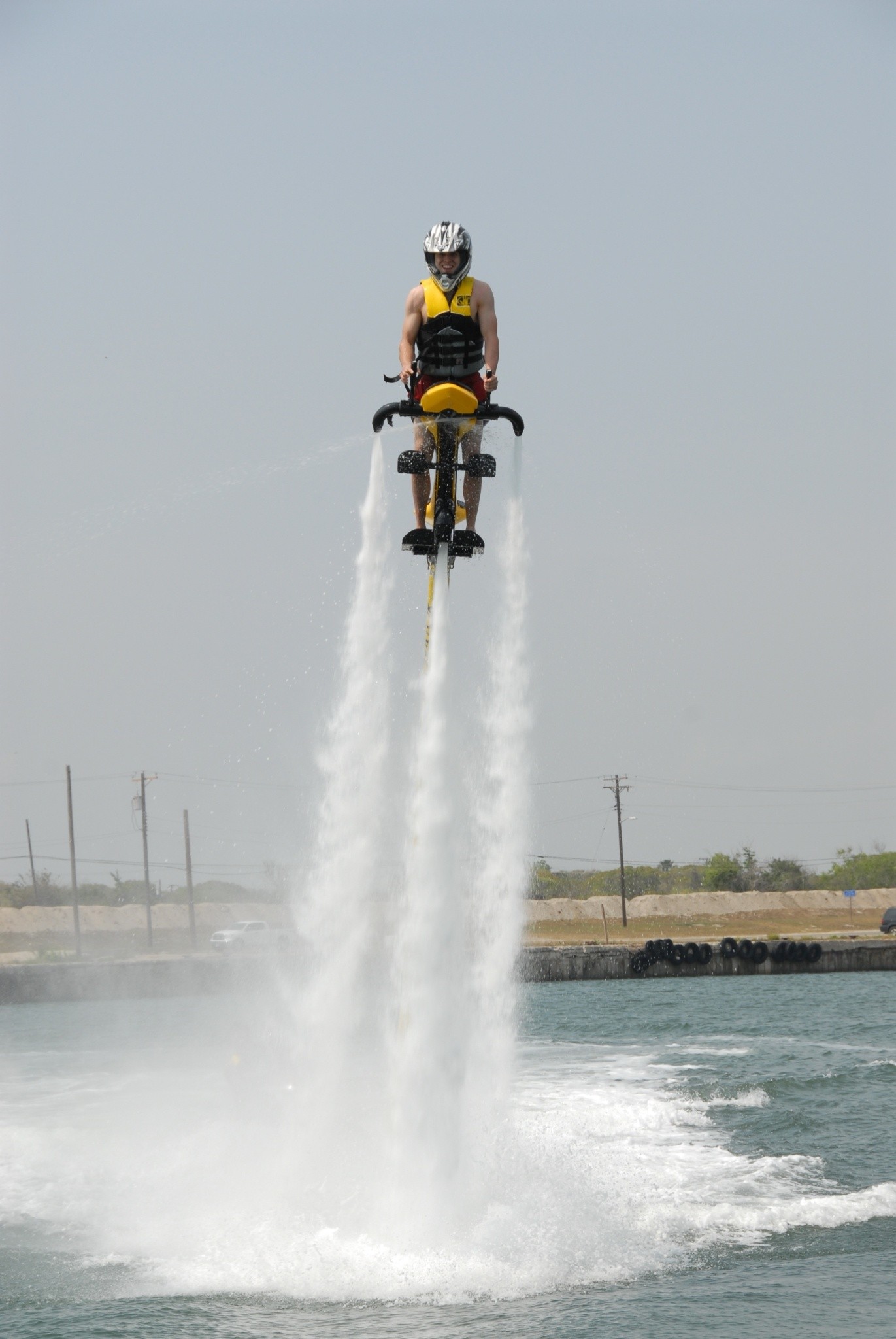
[400,222,499,533]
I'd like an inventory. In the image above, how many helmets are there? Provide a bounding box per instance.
[423,221,472,291]
